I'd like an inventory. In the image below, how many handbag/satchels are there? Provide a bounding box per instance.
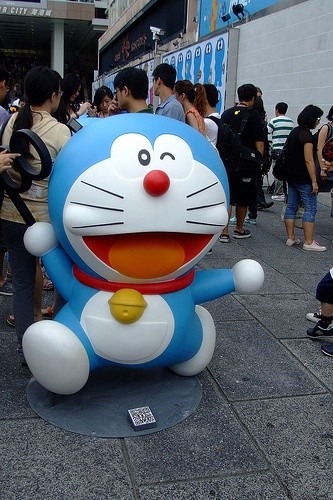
[281,196,306,222]
[234,157,259,177]
[272,128,317,181]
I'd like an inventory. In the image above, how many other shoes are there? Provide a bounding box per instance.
[0,268,57,368]
[305,312,321,322]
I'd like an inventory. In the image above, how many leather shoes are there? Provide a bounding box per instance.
[257,201,274,209]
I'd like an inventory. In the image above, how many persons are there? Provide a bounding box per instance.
[0,63,333,358]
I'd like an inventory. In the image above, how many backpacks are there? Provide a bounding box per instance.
[203,115,237,158]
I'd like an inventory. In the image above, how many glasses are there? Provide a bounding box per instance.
[113,89,121,94]
[55,91,64,96]
[2,81,10,91]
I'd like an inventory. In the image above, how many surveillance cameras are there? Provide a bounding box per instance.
[150,26,166,35]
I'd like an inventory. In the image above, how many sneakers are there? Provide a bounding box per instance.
[285,239,301,246]
[305,322,333,338]
[218,234,230,242]
[303,239,326,252]
[321,342,333,357]
[229,216,245,225]
[232,229,251,238]
[272,194,286,202]
[245,218,256,224]
